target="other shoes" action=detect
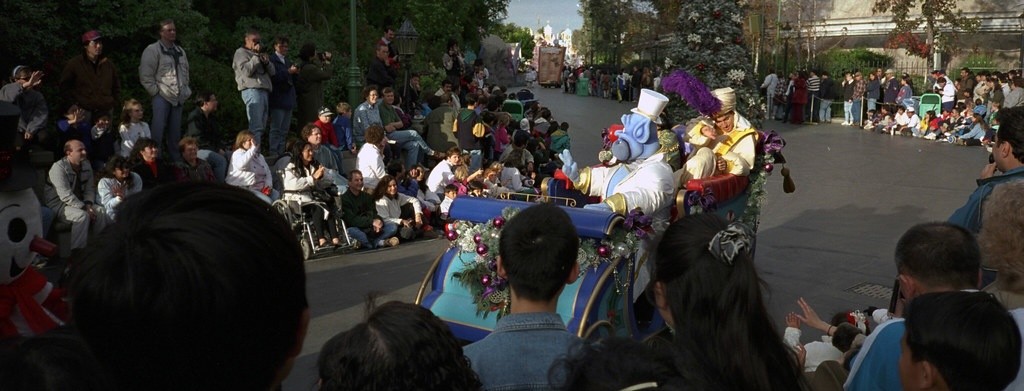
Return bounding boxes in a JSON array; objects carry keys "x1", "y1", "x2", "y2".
[
  {"x1": 825, "y1": 120, "x2": 831, "y2": 123},
  {"x1": 926, "y1": 133, "x2": 936, "y2": 140},
  {"x1": 841, "y1": 121, "x2": 848, "y2": 124},
  {"x1": 924, "y1": 132, "x2": 933, "y2": 138},
  {"x1": 632, "y1": 100, "x2": 637, "y2": 103},
  {"x1": 619, "y1": 99, "x2": 622, "y2": 103},
  {"x1": 848, "y1": 121, "x2": 853, "y2": 125},
  {"x1": 383, "y1": 237, "x2": 399, "y2": 247},
  {"x1": 331, "y1": 240, "x2": 339, "y2": 247},
  {"x1": 818, "y1": 121, "x2": 824, "y2": 124},
  {"x1": 319, "y1": 241, "x2": 333, "y2": 247}
]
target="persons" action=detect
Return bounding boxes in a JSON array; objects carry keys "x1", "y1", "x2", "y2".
[
  {"x1": 760, "y1": 68, "x2": 1024, "y2": 153},
  {"x1": 1, "y1": 107, "x2": 1024, "y2": 391},
  {"x1": 673, "y1": 87, "x2": 758, "y2": 190},
  {"x1": 0, "y1": 19, "x2": 571, "y2": 281},
  {"x1": 560, "y1": 64, "x2": 662, "y2": 104}
]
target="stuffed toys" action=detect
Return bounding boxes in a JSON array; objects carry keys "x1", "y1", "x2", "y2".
[
  {"x1": 558, "y1": 88, "x2": 685, "y2": 304},
  {"x1": 1, "y1": 101, "x2": 74, "y2": 339}
]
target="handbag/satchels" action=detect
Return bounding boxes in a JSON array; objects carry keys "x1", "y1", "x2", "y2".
[{"x1": 561, "y1": 85, "x2": 565, "y2": 93}]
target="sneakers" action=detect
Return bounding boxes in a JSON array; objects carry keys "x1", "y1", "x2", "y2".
[{"x1": 427, "y1": 149, "x2": 446, "y2": 160}]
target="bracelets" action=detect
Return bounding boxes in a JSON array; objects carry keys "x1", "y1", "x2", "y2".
[{"x1": 827, "y1": 325, "x2": 832, "y2": 336}]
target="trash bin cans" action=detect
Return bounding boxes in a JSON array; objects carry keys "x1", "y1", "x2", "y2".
[
  {"x1": 575, "y1": 78, "x2": 589, "y2": 96},
  {"x1": 919, "y1": 93, "x2": 941, "y2": 118},
  {"x1": 502, "y1": 100, "x2": 523, "y2": 123}
]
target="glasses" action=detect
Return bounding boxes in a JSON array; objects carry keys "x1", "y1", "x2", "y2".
[
  {"x1": 16, "y1": 77, "x2": 31, "y2": 82},
  {"x1": 115, "y1": 164, "x2": 129, "y2": 172}
]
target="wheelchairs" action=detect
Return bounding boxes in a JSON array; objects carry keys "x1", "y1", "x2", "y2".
[{"x1": 271, "y1": 190, "x2": 360, "y2": 261}]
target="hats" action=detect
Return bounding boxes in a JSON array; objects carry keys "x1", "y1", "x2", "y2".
[
  {"x1": 931, "y1": 69, "x2": 939, "y2": 73},
  {"x1": 663, "y1": 70, "x2": 737, "y2": 118},
  {"x1": 82, "y1": 31, "x2": 102, "y2": 42},
  {"x1": 885, "y1": 68, "x2": 894, "y2": 73},
  {"x1": 854, "y1": 72, "x2": 862, "y2": 75},
  {"x1": 318, "y1": 107, "x2": 334, "y2": 116},
  {"x1": 590, "y1": 67, "x2": 593, "y2": 69},
  {"x1": 905, "y1": 107, "x2": 914, "y2": 112}
]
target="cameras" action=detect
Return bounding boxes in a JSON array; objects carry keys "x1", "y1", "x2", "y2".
[
  {"x1": 318, "y1": 53, "x2": 333, "y2": 61},
  {"x1": 256, "y1": 42, "x2": 271, "y2": 53}
]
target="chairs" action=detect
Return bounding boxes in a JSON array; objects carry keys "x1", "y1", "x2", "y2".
[{"x1": 602, "y1": 124, "x2": 762, "y2": 217}]
